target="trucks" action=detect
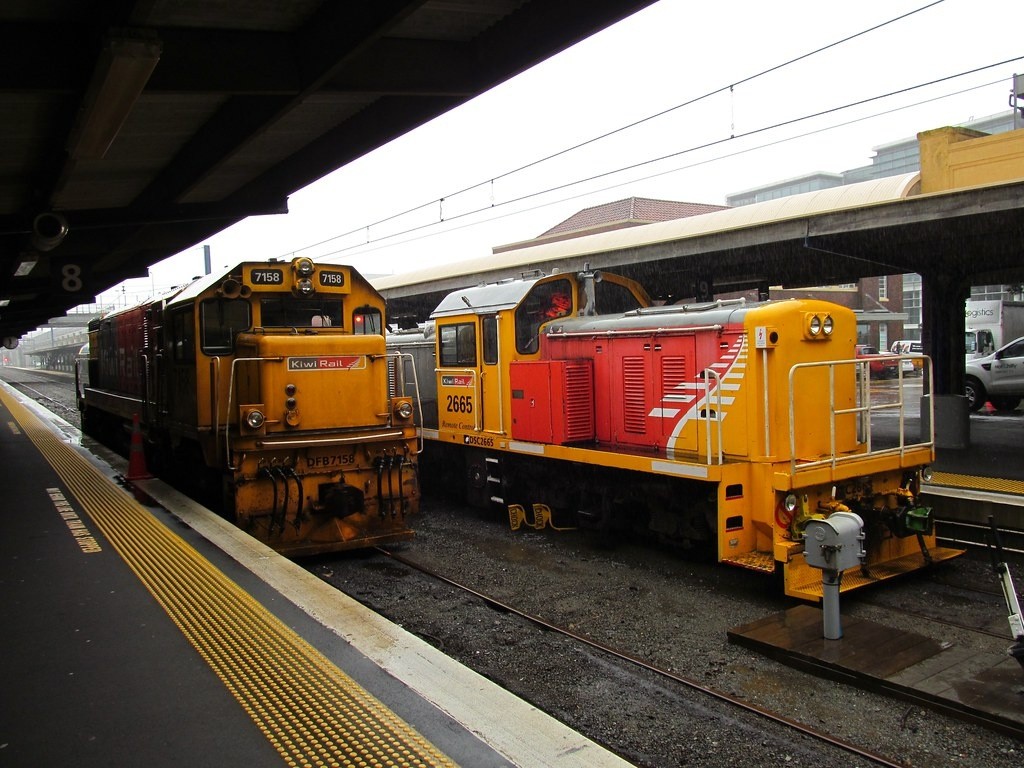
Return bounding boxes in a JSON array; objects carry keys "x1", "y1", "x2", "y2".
[{"x1": 965, "y1": 299, "x2": 1024, "y2": 360}]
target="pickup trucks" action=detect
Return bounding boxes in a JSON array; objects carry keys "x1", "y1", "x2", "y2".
[{"x1": 856, "y1": 340, "x2": 923, "y2": 376}]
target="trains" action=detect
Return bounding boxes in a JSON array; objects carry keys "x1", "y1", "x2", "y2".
[
  {"x1": 77, "y1": 264, "x2": 963, "y2": 603},
  {"x1": 74, "y1": 256, "x2": 424, "y2": 560}
]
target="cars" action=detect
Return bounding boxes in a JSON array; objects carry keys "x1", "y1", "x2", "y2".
[{"x1": 965, "y1": 336, "x2": 1024, "y2": 414}]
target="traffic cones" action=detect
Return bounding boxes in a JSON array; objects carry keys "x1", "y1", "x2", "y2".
[{"x1": 121, "y1": 413, "x2": 152, "y2": 480}]
